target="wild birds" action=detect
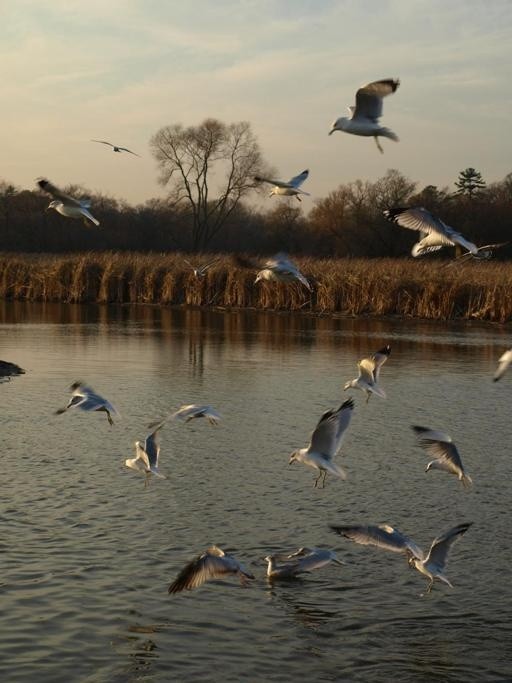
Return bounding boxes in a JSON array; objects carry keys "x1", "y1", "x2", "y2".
[
  {"x1": 288, "y1": 341, "x2": 393, "y2": 489},
  {"x1": 326, "y1": 522, "x2": 474, "y2": 592},
  {"x1": 254, "y1": 169, "x2": 311, "y2": 202},
  {"x1": 51, "y1": 378, "x2": 223, "y2": 488},
  {"x1": 181, "y1": 250, "x2": 322, "y2": 294},
  {"x1": 167, "y1": 546, "x2": 254, "y2": 593},
  {"x1": 37, "y1": 138, "x2": 138, "y2": 228},
  {"x1": 408, "y1": 423, "x2": 473, "y2": 490},
  {"x1": 327, "y1": 77, "x2": 401, "y2": 154},
  {"x1": 264, "y1": 546, "x2": 347, "y2": 581},
  {"x1": 491, "y1": 346, "x2": 512, "y2": 383},
  {"x1": 381, "y1": 206, "x2": 510, "y2": 269}
]
